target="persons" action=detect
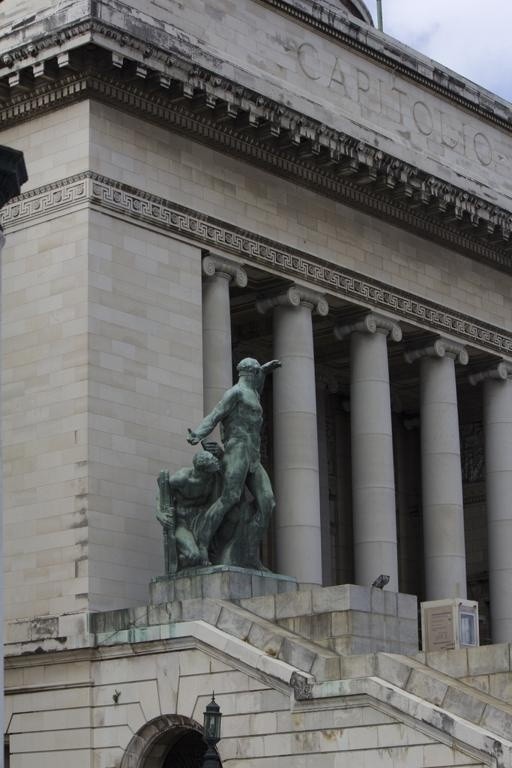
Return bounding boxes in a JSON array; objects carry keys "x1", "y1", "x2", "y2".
[
  {"x1": 143, "y1": 439, "x2": 222, "y2": 571},
  {"x1": 185, "y1": 354, "x2": 285, "y2": 577}
]
